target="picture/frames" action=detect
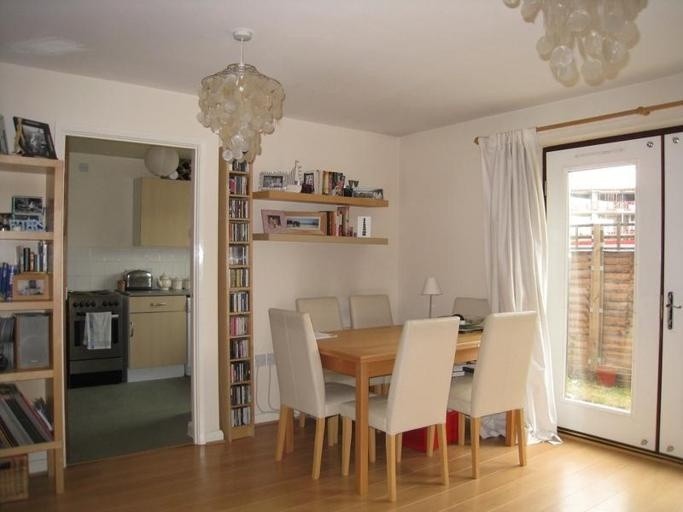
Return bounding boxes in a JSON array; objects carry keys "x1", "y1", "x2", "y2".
[
  {"x1": 282, "y1": 210, "x2": 327, "y2": 237},
  {"x1": 10, "y1": 272, "x2": 50, "y2": 302},
  {"x1": 11, "y1": 115, "x2": 57, "y2": 159},
  {"x1": 258, "y1": 170, "x2": 287, "y2": 192},
  {"x1": 260, "y1": 208, "x2": 287, "y2": 235}
]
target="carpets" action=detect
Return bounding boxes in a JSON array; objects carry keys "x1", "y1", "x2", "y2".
[{"x1": 64, "y1": 376, "x2": 195, "y2": 467}]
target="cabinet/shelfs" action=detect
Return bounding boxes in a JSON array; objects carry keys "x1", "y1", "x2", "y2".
[
  {"x1": 133, "y1": 175, "x2": 193, "y2": 250},
  {"x1": 249, "y1": 188, "x2": 390, "y2": 248},
  {"x1": 115, "y1": 290, "x2": 186, "y2": 383},
  {"x1": 216, "y1": 144, "x2": 255, "y2": 444},
  {"x1": 0, "y1": 151, "x2": 66, "y2": 506}
]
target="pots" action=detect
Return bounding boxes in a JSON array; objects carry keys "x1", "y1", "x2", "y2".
[{"x1": 123, "y1": 268, "x2": 154, "y2": 291}]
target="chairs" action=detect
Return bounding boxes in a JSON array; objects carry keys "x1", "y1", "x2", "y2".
[
  {"x1": 338, "y1": 314, "x2": 462, "y2": 505},
  {"x1": 450, "y1": 296, "x2": 491, "y2": 324},
  {"x1": 345, "y1": 292, "x2": 398, "y2": 436},
  {"x1": 423, "y1": 310, "x2": 542, "y2": 482},
  {"x1": 266, "y1": 305, "x2": 377, "y2": 484},
  {"x1": 293, "y1": 295, "x2": 366, "y2": 446}
]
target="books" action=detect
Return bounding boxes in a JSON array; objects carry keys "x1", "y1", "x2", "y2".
[
  {"x1": 303, "y1": 170, "x2": 346, "y2": 196},
  {"x1": 229, "y1": 244, "x2": 249, "y2": 265},
  {"x1": 229, "y1": 152, "x2": 248, "y2": 219},
  {"x1": 230, "y1": 223, "x2": 248, "y2": 242},
  {"x1": 231, "y1": 384, "x2": 252, "y2": 405},
  {"x1": 0, "y1": 241, "x2": 48, "y2": 301},
  {"x1": 0, "y1": 384, "x2": 53, "y2": 449},
  {"x1": 229, "y1": 269, "x2": 249, "y2": 287},
  {"x1": 231, "y1": 407, "x2": 250, "y2": 426},
  {"x1": 232, "y1": 292, "x2": 249, "y2": 312},
  {"x1": 230, "y1": 316, "x2": 248, "y2": 335},
  {"x1": 230, "y1": 338, "x2": 251, "y2": 382}
]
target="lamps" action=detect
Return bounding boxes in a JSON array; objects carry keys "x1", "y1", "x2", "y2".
[
  {"x1": 144, "y1": 148, "x2": 181, "y2": 177},
  {"x1": 501, "y1": 0, "x2": 653, "y2": 89},
  {"x1": 194, "y1": 28, "x2": 288, "y2": 165},
  {"x1": 419, "y1": 275, "x2": 442, "y2": 321}
]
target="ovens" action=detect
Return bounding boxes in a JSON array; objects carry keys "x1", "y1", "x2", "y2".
[{"x1": 65, "y1": 288, "x2": 125, "y2": 389}]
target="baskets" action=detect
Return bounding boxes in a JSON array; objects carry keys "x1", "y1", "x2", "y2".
[{"x1": 0, "y1": 453, "x2": 33, "y2": 505}]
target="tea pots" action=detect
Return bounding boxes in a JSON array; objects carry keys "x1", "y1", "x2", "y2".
[{"x1": 158, "y1": 272, "x2": 193, "y2": 291}]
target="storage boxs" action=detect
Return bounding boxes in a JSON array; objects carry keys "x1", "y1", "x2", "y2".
[{"x1": 401, "y1": 409, "x2": 460, "y2": 454}]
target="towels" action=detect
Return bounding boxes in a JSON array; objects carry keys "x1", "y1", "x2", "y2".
[{"x1": 82, "y1": 311, "x2": 113, "y2": 351}]
